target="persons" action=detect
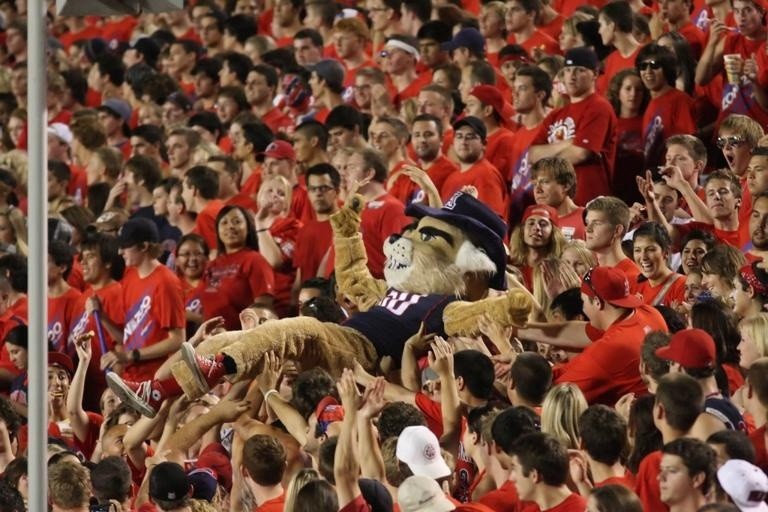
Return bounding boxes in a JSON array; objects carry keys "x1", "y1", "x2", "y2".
[{"x1": 0, "y1": 0, "x2": 767, "y2": 511}]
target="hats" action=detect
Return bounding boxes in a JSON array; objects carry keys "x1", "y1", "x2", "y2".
[
  {"x1": 580, "y1": 266, "x2": 643, "y2": 309},
  {"x1": 453, "y1": 83, "x2": 508, "y2": 142},
  {"x1": 564, "y1": 46, "x2": 600, "y2": 72},
  {"x1": 302, "y1": 59, "x2": 345, "y2": 88},
  {"x1": 47, "y1": 97, "x2": 131, "y2": 149},
  {"x1": 396, "y1": 425, "x2": 452, "y2": 480},
  {"x1": 254, "y1": 138, "x2": 296, "y2": 163},
  {"x1": 438, "y1": 27, "x2": 485, "y2": 53},
  {"x1": 655, "y1": 328, "x2": 718, "y2": 368},
  {"x1": 46, "y1": 351, "x2": 73, "y2": 370},
  {"x1": 717, "y1": 458, "x2": 768, "y2": 512},
  {"x1": 148, "y1": 440, "x2": 234, "y2": 503},
  {"x1": 397, "y1": 475, "x2": 456, "y2": 512},
  {"x1": 90, "y1": 205, "x2": 160, "y2": 249}
]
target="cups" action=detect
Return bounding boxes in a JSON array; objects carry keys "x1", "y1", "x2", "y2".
[{"x1": 723, "y1": 53, "x2": 741, "y2": 85}]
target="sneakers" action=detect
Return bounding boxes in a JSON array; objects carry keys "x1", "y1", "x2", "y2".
[
  {"x1": 104, "y1": 372, "x2": 168, "y2": 420},
  {"x1": 180, "y1": 341, "x2": 227, "y2": 391}
]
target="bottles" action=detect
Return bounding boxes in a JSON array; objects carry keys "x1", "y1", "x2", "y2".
[{"x1": 74, "y1": 187, "x2": 82, "y2": 208}]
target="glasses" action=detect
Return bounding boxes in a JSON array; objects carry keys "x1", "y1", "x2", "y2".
[
  {"x1": 637, "y1": 59, "x2": 665, "y2": 70},
  {"x1": 716, "y1": 134, "x2": 748, "y2": 149},
  {"x1": 583, "y1": 268, "x2": 604, "y2": 304}
]
